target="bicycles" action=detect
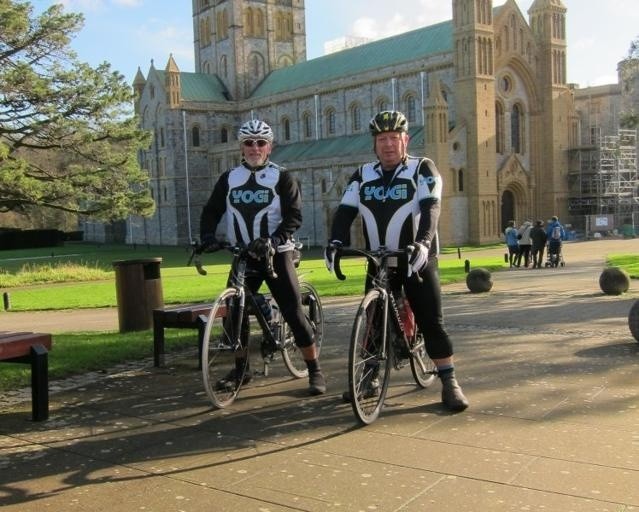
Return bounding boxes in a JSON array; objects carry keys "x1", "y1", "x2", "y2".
[
  {"x1": 185, "y1": 237, "x2": 324, "y2": 409},
  {"x1": 327, "y1": 238, "x2": 441, "y2": 426}
]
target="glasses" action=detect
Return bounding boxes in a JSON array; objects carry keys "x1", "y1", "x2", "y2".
[{"x1": 244, "y1": 140, "x2": 266, "y2": 146}]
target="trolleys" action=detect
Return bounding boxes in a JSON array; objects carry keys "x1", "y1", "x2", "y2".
[{"x1": 545, "y1": 243, "x2": 565, "y2": 268}]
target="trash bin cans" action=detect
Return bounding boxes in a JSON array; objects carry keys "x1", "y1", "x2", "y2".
[{"x1": 111, "y1": 257, "x2": 163, "y2": 332}]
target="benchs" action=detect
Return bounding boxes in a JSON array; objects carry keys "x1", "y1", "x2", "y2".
[
  {"x1": 0, "y1": 329, "x2": 52, "y2": 421},
  {"x1": 151, "y1": 291, "x2": 317, "y2": 369}
]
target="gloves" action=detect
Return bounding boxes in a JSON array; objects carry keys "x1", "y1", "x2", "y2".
[
  {"x1": 200, "y1": 233, "x2": 220, "y2": 253},
  {"x1": 249, "y1": 238, "x2": 273, "y2": 255},
  {"x1": 324, "y1": 239, "x2": 344, "y2": 271},
  {"x1": 408, "y1": 240, "x2": 431, "y2": 272}
]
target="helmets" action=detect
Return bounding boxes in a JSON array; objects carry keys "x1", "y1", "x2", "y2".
[
  {"x1": 368, "y1": 110, "x2": 409, "y2": 137},
  {"x1": 237, "y1": 120, "x2": 274, "y2": 143}
]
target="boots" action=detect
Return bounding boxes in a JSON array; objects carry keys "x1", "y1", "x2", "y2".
[
  {"x1": 438, "y1": 368, "x2": 469, "y2": 410},
  {"x1": 305, "y1": 358, "x2": 326, "y2": 394},
  {"x1": 216, "y1": 355, "x2": 251, "y2": 389},
  {"x1": 343, "y1": 363, "x2": 380, "y2": 403}
]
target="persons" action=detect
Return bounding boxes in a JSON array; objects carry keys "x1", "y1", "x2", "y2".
[
  {"x1": 323, "y1": 110, "x2": 470, "y2": 413},
  {"x1": 199, "y1": 120, "x2": 326, "y2": 397},
  {"x1": 503, "y1": 214, "x2": 565, "y2": 269}
]
